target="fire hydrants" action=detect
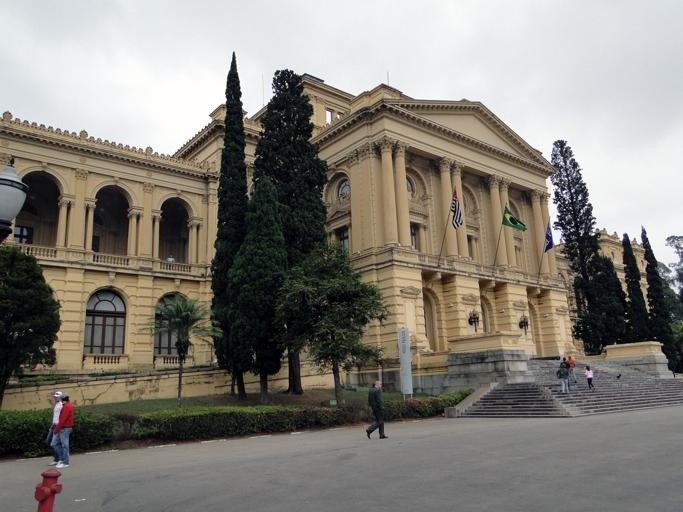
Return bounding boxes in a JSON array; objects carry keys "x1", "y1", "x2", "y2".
[{"x1": 34, "y1": 468, "x2": 63, "y2": 512}]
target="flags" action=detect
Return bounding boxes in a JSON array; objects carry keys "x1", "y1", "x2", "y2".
[
  {"x1": 501, "y1": 206, "x2": 528, "y2": 232},
  {"x1": 543, "y1": 224, "x2": 553, "y2": 253},
  {"x1": 449, "y1": 190, "x2": 463, "y2": 230}
]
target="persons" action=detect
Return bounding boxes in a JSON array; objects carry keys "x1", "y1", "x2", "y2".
[
  {"x1": 584, "y1": 366, "x2": 595, "y2": 392},
  {"x1": 50, "y1": 393, "x2": 74, "y2": 468},
  {"x1": 558, "y1": 356, "x2": 577, "y2": 393},
  {"x1": 46, "y1": 391, "x2": 63, "y2": 466},
  {"x1": 165, "y1": 254, "x2": 175, "y2": 270},
  {"x1": 366, "y1": 381, "x2": 389, "y2": 439}
]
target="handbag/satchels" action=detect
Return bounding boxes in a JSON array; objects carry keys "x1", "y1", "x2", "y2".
[{"x1": 556, "y1": 370, "x2": 561, "y2": 378}]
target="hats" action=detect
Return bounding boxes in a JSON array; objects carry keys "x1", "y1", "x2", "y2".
[{"x1": 52, "y1": 391, "x2": 69, "y2": 398}]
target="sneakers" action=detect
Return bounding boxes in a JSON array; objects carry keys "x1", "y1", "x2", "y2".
[
  {"x1": 575, "y1": 380, "x2": 577, "y2": 383},
  {"x1": 48, "y1": 461, "x2": 69, "y2": 468}
]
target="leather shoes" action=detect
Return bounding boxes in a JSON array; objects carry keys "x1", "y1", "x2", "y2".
[
  {"x1": 380, "y1": 436, "x2": 388, "y2": 439},
  {"x1": 366, "y1": 430, "x2": 370, "y2": 439}
]
